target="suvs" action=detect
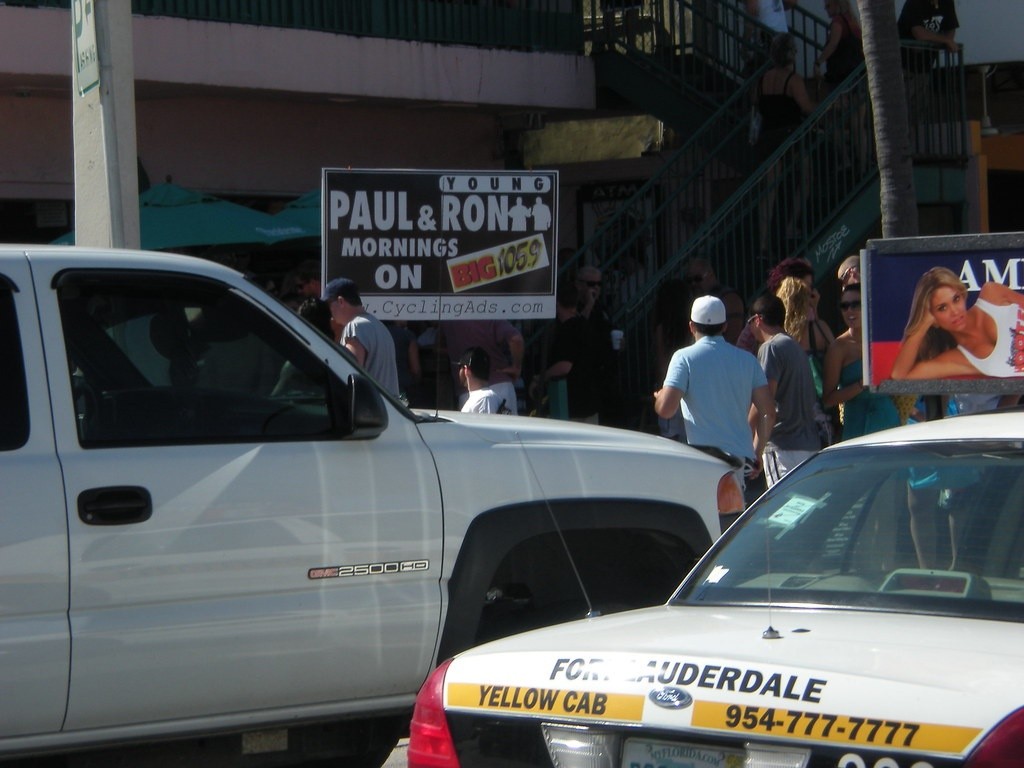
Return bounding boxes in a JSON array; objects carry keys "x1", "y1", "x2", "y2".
[{"x1": 1, "y1": 245, "x2": 755, "y2": 765}]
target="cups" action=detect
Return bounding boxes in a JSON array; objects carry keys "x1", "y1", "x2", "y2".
[{"x1": 610, "y1": 330, "x2": 624, "y2": 350}]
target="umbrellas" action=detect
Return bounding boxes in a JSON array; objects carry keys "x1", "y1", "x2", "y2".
[
  {"x1": 273, "y1": 186, "x2": 321, "y2": 232},
  {"x1": 49, "y1": 183, "x2": 321, "y2": 250}
]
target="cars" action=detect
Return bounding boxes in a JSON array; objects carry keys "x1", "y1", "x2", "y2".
[{"x1": 407, "y1": 411, "x2": 1024, "y2": 768}]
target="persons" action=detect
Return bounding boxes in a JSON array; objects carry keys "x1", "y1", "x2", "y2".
[
  {"x1": 740, "y1": 0, "x2": 959, "y2": 257},
  {"x1": 651, "y1": 298, "x2": 693, "y2": 442},
  {"x1": 837, "y1": 255, "x2": 860, "y2": 288},
  {"x1": 907, "y1": 391, "x2": 1024, "y2": 572},
  {"x1": 320, "y1": 278, "x2": 399, "y2": 398},
  {"x1": 270, "y1": 299, "x2": 336, "y2": 397},
  {"x1": 822, "y1": 283, "x2": 902, "y2": 441},
  {"x1": 600, "y1": 0, "x2": 644, "y2": 51},
  {"x1": 891, "y1": 266, "x2": 1024, "y2": 381},
  {"x1": 451, "y1": 346, "x2": 511, "y2": 414},
  {"x1": 233, "y1": 259, "x2": 626, "y2": 427},
  {"x1": 748, "y1": 295, "x2": 821, "y2": 488},
  {"x1": 654, "y1": 295, "x2": 777, "y2": 509},
  {"x1": 736, "y1": 258, "x2": 814, "y2": 358},
  {"x1": 776, "y1": 276, "x2": 843, "y2": 450},
  {"x1": 687, "y1": 257, "x2": 745, "y2": 345}
]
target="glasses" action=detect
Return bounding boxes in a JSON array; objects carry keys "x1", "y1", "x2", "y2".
[
  {"x1": 586, "y1": 280, "x2": 602, "y2": 286},
  {"x1": 840, "y1": 300, "x2": 861, "y2": 311}
]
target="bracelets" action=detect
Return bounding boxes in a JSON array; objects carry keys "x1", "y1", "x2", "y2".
[{"x1": 858, "y1": 381, "x2": 865, "y2": 392}]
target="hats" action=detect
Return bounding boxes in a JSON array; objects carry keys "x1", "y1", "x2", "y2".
[
  {"x1": 691, "y1": 294, "x2": 726, "y2": 325},
  {"x1": 557, "y1": 286, "x2": 580, "y2": 307},
  {"x1": 460, "y1": 347, "x2": 491, "y2": 379},
  {"x1": 318, "y1": 277, "x2": 359, "y2": 301}
]
